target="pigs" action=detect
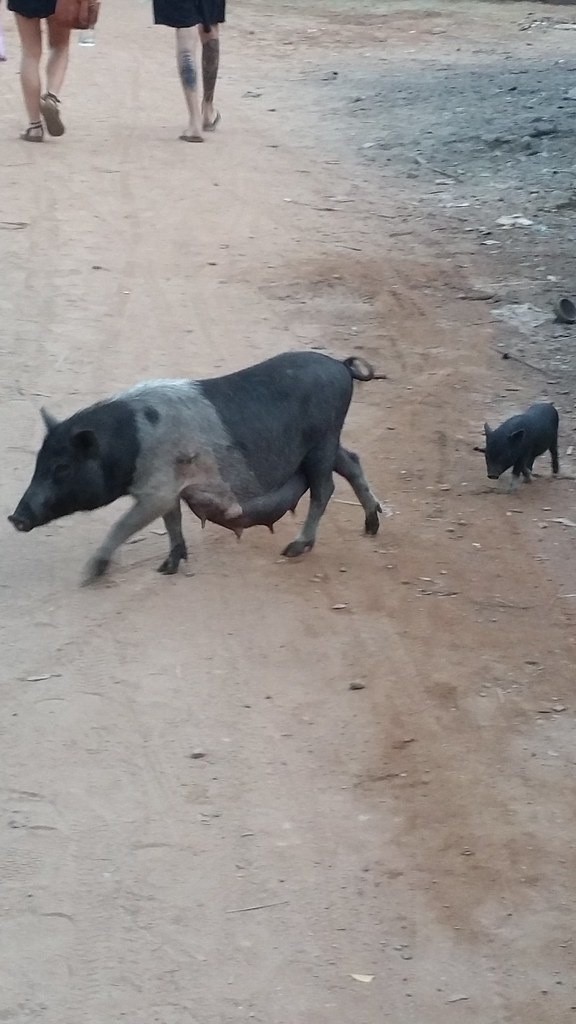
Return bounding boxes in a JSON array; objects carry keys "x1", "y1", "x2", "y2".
[
  {"x1": 484, "y1": 402, "x2": 559, "y2": 486},
  {"x1": 6, "y1": 351, "x2": 381, "y2": 587}
]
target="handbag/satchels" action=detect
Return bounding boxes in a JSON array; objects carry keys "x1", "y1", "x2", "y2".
[{"x1": 54, "y1": 1, "x2": 100, "y2": 29}]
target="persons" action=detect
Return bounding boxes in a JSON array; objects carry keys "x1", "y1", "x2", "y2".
[
  {"x1": 151, "y1": 0, "x2": 228, "y2": 143},
  {"x1": 4, "y1": 0, "x2": 73, "y2": 144}
]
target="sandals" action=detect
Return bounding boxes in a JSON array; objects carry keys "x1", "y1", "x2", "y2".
[
  {"x1": 40, "y1": 93, "x2": 65, "y2": 137},
  {"x1": 20, "y1": 120, "x2": 45, "y2": 142}
]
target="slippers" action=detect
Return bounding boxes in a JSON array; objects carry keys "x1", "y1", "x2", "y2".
[
  {"x1": 203, "y1": 109, "x2": 222, "y2": 131},
  {"x1": 179, "y1": 128, "x2": 204, "y2": 143}
]
